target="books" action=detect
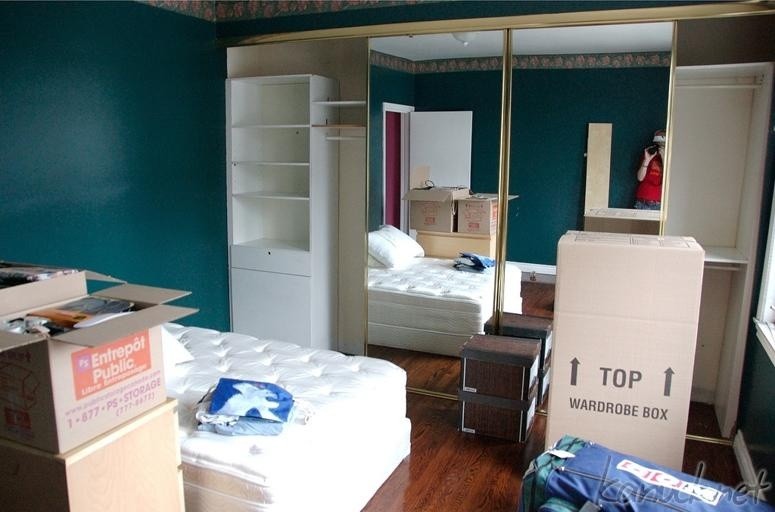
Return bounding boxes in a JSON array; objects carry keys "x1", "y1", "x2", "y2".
[{"x1": 24, "y1": 296, "x2": 136, "y2": 328}]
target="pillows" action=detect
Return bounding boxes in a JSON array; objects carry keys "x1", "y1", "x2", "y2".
[{"x1": 366, "y1": 223, "x2": 426, "y2": 271}]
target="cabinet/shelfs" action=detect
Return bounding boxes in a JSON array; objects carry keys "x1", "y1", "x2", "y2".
[
  {"x1": 224, "y1": 73, "x2": 367, "y2": 350},
  {"x1": 411, "y1": 229, "x2": 499, "y2": 261},
  {"x1": 1, "y1": 395, "x2": 189, "y2": 512},
  {"x1": 663, "y1": 57, "x2": 774, "y2": 442}
]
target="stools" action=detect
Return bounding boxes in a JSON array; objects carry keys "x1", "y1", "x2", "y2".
[
  {"x1": 483, "y1": 307, "x2": 553, "y2": 410},
  {"x1": 456, "y1": 330, "x2": 542, "y2": 443}
]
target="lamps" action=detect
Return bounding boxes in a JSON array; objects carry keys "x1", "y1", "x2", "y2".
[{"x1": 452, "y1": 31, "x2": 479, "y2": 46}]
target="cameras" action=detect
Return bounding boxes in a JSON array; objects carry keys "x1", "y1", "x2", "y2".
[{"x1": 648, "y1": 143, "x2": 660, "y2": 155}]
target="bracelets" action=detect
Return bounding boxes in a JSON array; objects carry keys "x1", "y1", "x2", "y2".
[{"x1": 641, "y1": 164, "x2": 648, "y2": 169}]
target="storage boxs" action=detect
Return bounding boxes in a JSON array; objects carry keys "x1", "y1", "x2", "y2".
[
  {"x1": 0, "y1": 253, "x2": 129, "y2": 320},
  {"x1": 544, "y1": 228, "x2": 707, "y2": 471},
  {"x1": 0, "y1": 282, "x2": 201, "y2": 454},
  {"x1": 452, "y1": 191, "x2": 522, "y2": 236},
  {"x1": 399, "y1": 181, "x2": 470, "y2": 233}
]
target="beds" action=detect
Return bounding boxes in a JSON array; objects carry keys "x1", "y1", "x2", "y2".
[
  {"x1": 367, "y1": 222, "x2": 525, "y2": 359},
  {"x1": 159, "y1": 322, "x2": 414, "y2": 512}
]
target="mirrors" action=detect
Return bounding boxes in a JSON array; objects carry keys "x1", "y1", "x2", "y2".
[{"x1": 367, "y1": 18, "x2": 679, "y2": 418}]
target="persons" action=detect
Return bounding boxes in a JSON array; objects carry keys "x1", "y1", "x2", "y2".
[{"x1": 634, "y1": 127, "x2": 667, "y2": 210}]
